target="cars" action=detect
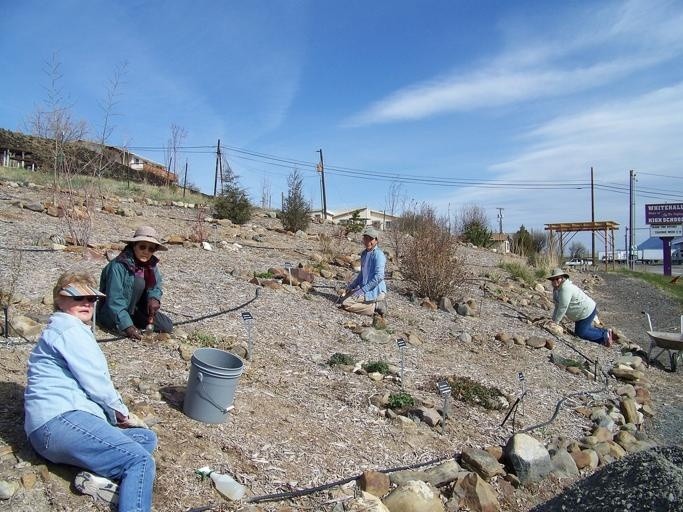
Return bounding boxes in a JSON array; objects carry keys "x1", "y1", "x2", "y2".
[{"x1": 565, "y1": 258, "x2": 593, "y2": 266}]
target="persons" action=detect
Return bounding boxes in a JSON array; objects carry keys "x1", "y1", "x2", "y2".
[
  {"x1": 21, "y1": 266, "x2": 158, "y2": 512},
  {"x1": 542, "y1": 267, "x2": 613, "y2": 348},
  {"x1": 336, "y1": 226, "x2": 389, "y2": 320},
  {"x1": 88, "y1": 226, "x2": 174, "y2": 344}
]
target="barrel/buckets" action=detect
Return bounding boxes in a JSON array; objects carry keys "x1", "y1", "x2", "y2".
[{"x1": 183, "y1": 347, "x2": 244, "y2": 423}]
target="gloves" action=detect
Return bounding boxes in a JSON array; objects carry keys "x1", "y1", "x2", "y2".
[
  {"x1": 125, "y1": 325, "x2": 141, "y2": 341},
  {"x1": 146, "y1": 298, "x2": 160, "y2": 315},
  {"x1": 116, "y1": 413, "x2": 148, "y2": 429},
  {"x1": 345, "y1": 286, "x2": 353, "y2": 293},
  {"x1": 351, "y1": 288, "x2": 364, "y2": 302}
]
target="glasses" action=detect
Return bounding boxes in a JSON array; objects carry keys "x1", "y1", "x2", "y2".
[
  {"x1": 73, "y1": 296, "x2": 97, "y2": 302},
  {"x1": 138, "y1": 245, "x2": 155, "y2": 252},
  {"x1": 551, "y1": 277, "x2": 559, "y2": 282}
]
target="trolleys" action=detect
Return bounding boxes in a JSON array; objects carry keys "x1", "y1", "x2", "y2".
[{"x1": 640, "y1": 310, "x2": 683, "y2": 372}]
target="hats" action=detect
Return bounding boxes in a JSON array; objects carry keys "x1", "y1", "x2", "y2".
[
  {"x1": 360, "y1": 229, "x2": 378, "y2": 240},
  {"x1": 545, "y1": 268, "x2": 568, "y2": 281},
  {"x1": 58, "y1": 282, "x2": 106, "y2": 297},
  {"x1": 118, "y1": 226, "x2": 168, "y2": 250}
]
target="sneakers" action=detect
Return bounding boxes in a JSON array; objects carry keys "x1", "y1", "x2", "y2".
[
  {"x1": 375, "y1": 302, "x2": 386, "y2": 320},
  {"x1": 74, "y1": 471, "x2": 120, "y2": 505},
  {"x1": 606, "y1": 328, "x2": 612, "y2": 347}
]
